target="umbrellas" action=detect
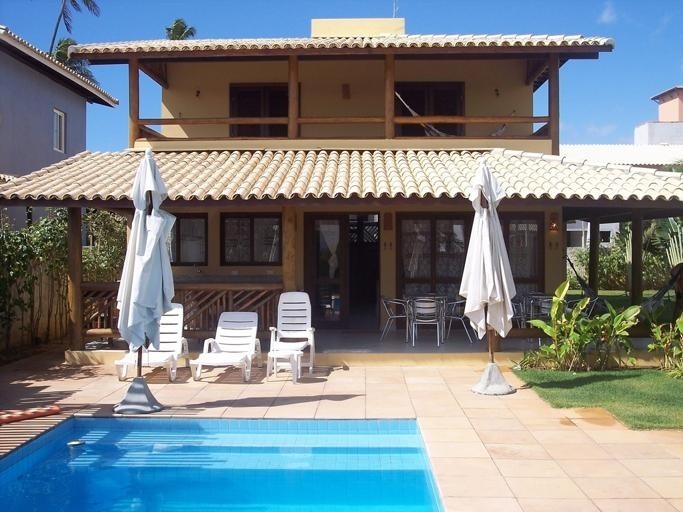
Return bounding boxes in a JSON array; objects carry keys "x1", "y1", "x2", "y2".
[
  {"x1": 114, "y1": 145, "x2": 177, "y2": 378},
  {"x1": 455, "y1": 154, "x2": 518, "y2": 363}
]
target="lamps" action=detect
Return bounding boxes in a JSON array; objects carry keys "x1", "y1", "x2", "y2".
[{"x1": 549, "y1": 213, "x2": 559, "y2": 233}]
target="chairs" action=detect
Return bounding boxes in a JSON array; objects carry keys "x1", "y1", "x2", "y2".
[
  {"x1": 379, "y1": 294, "x2": 473, "y2": 348},
  {"x1": 512, "y1": 292, "x2": 598, "y2": 329},
  {"x1": 114, "y1": 291, "x2": 315, "y2": 385}
]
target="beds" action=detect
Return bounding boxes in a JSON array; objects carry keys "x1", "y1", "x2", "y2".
[
  {"x1": 568, "y1": 254, "x2": 683, "y2": 317},
  {"x1": 395, "y1": 91, "x2": 519, "y2": 138}
]
